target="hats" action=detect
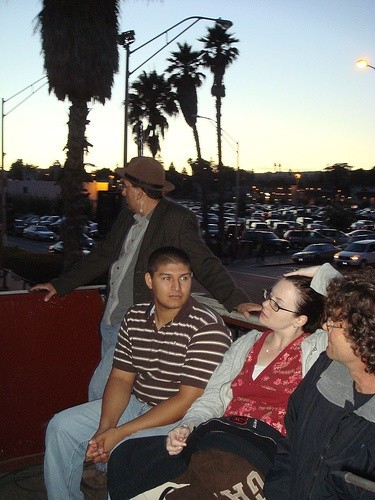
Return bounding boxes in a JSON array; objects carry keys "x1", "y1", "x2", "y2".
[{"x1": 114, "y1": 156, "x2": 175, "y2": 191}]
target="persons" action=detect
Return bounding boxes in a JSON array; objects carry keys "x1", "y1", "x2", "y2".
[
  {"x1": 28, "y1": 156, "x2": 263, "y2": 492},
  {"x1": 262, "y1": 270, "x2": 375, "y2": 500},
  {"x1": 106, "y1": 262, "x2": 346, "y2": 500},
  {"x1": 43, "y1": 245, "x2": 235, "y2": 500}
]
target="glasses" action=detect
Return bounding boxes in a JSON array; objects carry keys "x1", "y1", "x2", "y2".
[
  {"x1": 263, "y1": 288, "x2": 303, "y2": 315},
  {"x1": 326, "y1": 317, "x2": 349, "y2": 333},
  {"x1": 121, "y1": 185, "x2": 142, "y2": 189}
]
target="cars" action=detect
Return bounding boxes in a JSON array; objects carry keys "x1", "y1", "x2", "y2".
[
  {"x1": 290, "y1": 243, "x2": 336, "y2": 266},
  {"x1": 13, "y1": 213, "x2": 100, "y2": 255},
  {"x1": 314, "y1": 228, "x2": 350, "y2": 243},
  {"x1": 174, "y1": 201, "x2": 375, "y2": 243},
  {"x1": 239, "y1": 230, "x2": 290, "y2": 255},
  {"x1": 333, "y1": 239, "x2": 375, "y2": 269},
  {"x1": 23, "y1": 225, "x2": 57, "y2": 241},
  {"x1": 283, "y1": 230, "x2": 332, "y2": 245}
]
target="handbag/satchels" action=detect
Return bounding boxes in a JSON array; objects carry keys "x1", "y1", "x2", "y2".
[
  {"x1": 182, "y1": 415, "x2": 290, "y2": 483},
  {"x1": 158, "y1": 449, "x2": 267, "y2": 500}
]
